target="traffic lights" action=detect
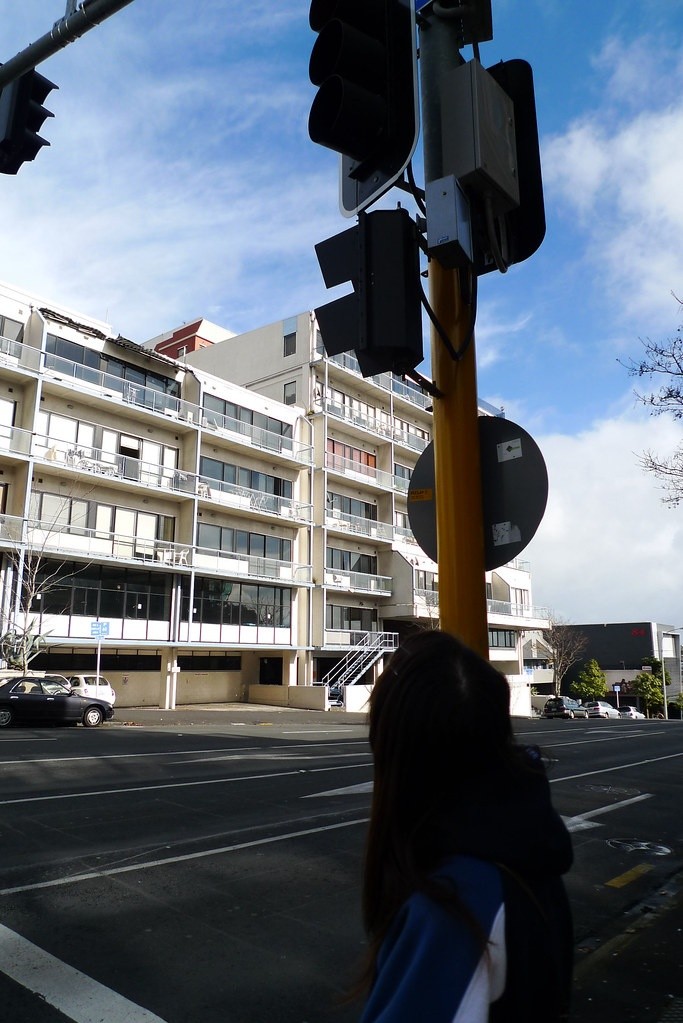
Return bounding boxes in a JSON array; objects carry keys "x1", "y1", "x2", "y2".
[
  {"x1": 303, "y1": 1, "x2": 419, "y2": 219},
  {"x1": 0, "y1": 63, "x2": 55, "y2": 175},
  {"x1": 461, "y1": 56, "x2": 547, "y2": 277},
  {"x1": 312, "y1": 205, "x2": 425, "y2": 374}
]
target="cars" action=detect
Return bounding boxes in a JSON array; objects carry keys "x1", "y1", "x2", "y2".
[
  {"x1": 616, "y1": 706, "x2": 646, "y2": 720},
  {"x1": 543, "y1": 698, "x2": 589, "y2": 719},
  {"x1": 583, "y1": 701, "x2": 623, "y2": 718},
  {"x1": 66, "y1": 675, "x2": 115, "y2": 706},
  {"x1": 43, "y1": 673, "x2": 71, "y2": 696},
  {"x1": 0, "y1": 677, "x2": 114, "y2": 727}
]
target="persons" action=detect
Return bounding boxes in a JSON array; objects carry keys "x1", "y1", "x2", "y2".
[{"x1": 349, "y1": 632, "x2": 580, "y2": 1022}]
